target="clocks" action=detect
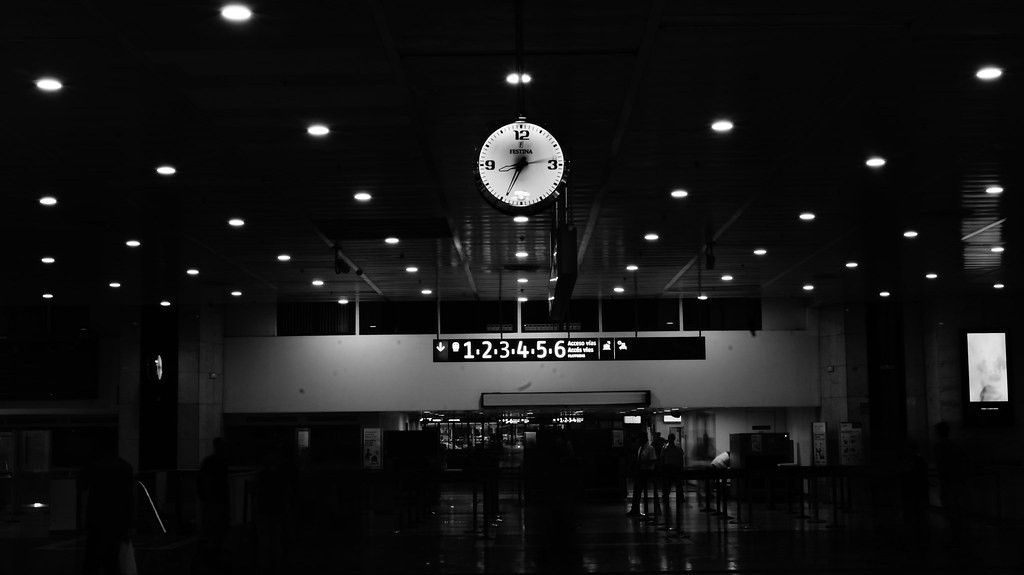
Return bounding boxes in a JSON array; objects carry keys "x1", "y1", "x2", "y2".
[{"x1": 471, "y1": 114, "x2": 571, "y2": 216}]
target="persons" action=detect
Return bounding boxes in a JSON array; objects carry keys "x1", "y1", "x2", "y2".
[
  {"x1": 196, "y1": 437, "x2": 232, "y2": 561},
  {"x1": 933, "y1": 423, "x2": 971, "y2": 547},
  {"x1": 79, "y1": 435, "x2": 134, "y2": 575},
  {"x1": 895, "y1": 440, "x2": 930, "y2": 551},
  {"x1": 626, "y1": 432, "x2": 687, "y2": 537}
]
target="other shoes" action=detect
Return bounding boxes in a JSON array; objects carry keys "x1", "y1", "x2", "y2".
[{"x1": 625, "y1": 511, "x2": 641, "y2": 516}]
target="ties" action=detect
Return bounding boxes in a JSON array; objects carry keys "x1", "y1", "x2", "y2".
[{"x1": 639, "y1": 447, "x2": 644, "y2": 461}]
